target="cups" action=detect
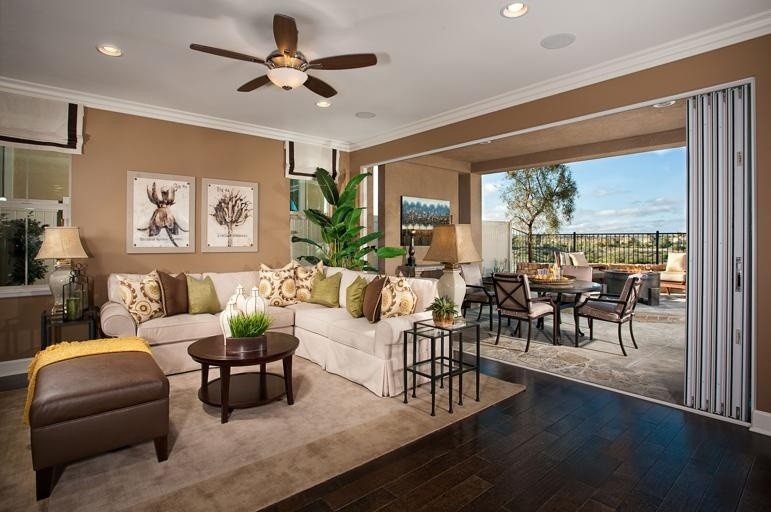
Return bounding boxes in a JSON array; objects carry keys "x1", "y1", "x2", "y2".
[{"x1": 537, "y1": 264, "x2": 564, "y2": 282}]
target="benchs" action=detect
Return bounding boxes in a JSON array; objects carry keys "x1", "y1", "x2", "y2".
[{"x1": 27, "y1": 340, "x2": 174, "y2": 503}]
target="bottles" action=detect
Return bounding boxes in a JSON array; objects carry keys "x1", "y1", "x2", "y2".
[{"x1": 219, "y1": 284, "x2": 266, "y2": 347}]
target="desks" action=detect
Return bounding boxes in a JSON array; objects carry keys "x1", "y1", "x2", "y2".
[
  {"x1": 184, "y1": 329, "x2": 302, "y2": 425},
  {"x1": 400, "y1": 316, "x2": 483, "y2": 418},
  {"x1": 37, "y1": 308, "x2": 101, "y2": 349}
]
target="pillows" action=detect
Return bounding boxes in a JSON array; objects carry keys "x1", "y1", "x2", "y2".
[
  {"x1": 256, "y1": 257, "x2": 420, "y2": 326},
  {"x1": 114, "y1": 259, "x2": 226, "y2": 322}
]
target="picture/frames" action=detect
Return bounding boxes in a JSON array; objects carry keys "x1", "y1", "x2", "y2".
[
  {"x1": 200, "y1": 176, "x2": 261, "y2": 254},
  {"x1": 124, "y1": 169, "x2": 198, "y2": 254}
]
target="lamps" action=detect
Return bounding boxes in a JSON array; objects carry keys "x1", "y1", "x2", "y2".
[
  {"x1": 422, "y1": 223, "x2": 486, "y2": 322},
  {"x1": 263, "y1": 62, "x2": 311, "y2": 91},
  {"x1": 33, "y1": 226, "x2": 95, "y2": 318}
]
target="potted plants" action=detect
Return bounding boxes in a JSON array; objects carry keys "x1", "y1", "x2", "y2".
[
  {"x1": 423, "y1": 295, "x2": 462, "y2": 326},
  {"x1": 225, "y1": 308, "x2": 272, "y2": 359}
]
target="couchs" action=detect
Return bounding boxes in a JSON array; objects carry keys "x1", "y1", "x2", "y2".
[{"x1": 98, "y1": 257, "x2": 463, "y2": 402}]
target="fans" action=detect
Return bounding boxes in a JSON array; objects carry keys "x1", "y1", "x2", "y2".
[{"x1": 187, "y1": 12, "x2": 379, "y2": 98}]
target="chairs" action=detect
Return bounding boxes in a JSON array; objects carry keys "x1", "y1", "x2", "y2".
[{"x1": 459, "y1": 244, "x2": 689, "y2": 360}]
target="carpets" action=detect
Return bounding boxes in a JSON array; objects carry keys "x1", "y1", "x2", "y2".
[{"x1": 1, "y1": 340, "x2": 532, "y2": 511}]
